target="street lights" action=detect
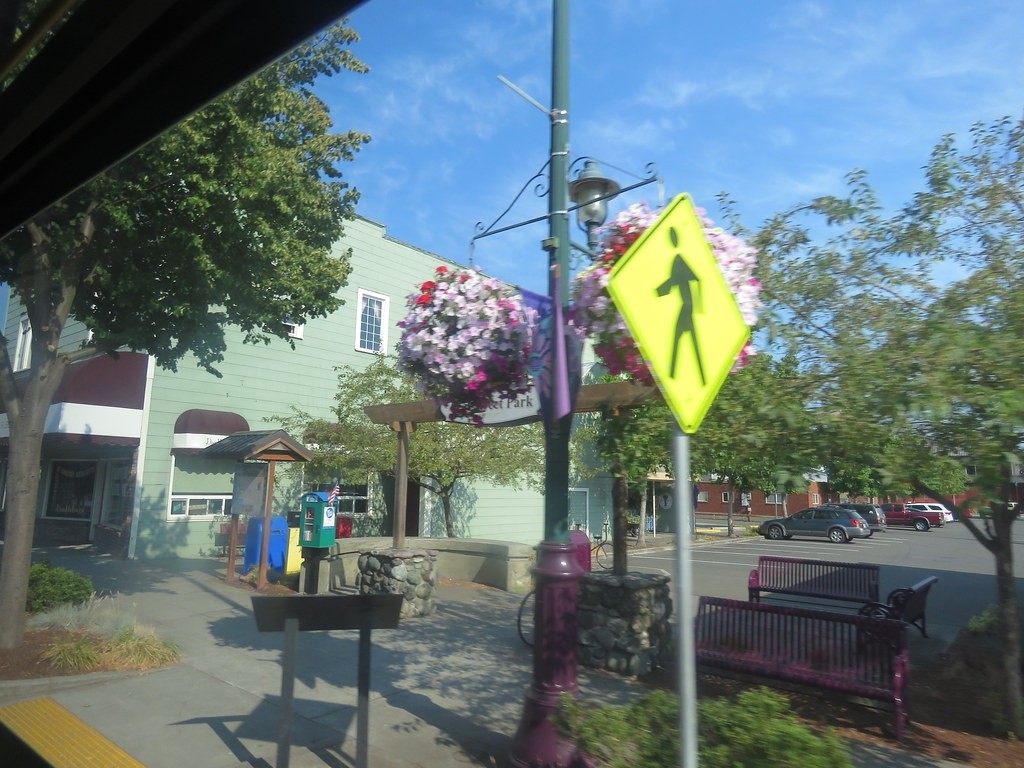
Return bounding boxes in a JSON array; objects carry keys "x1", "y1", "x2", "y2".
[{"x1": 468, "y1": 0, "x2": 660, "y2": 768}]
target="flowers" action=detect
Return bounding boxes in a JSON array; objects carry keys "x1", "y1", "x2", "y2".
[
  {"x1": 394, "y1": 266, "x2": 537, "y2": 428},
  {"x1": 567, "y1": 202, "x2": 763, "y2": 399}
]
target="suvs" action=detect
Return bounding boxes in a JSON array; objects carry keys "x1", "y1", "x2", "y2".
[{"x1": 826, "y1": 503, "x2": 887, "y2": 535}]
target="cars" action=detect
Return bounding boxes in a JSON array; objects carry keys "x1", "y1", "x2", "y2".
[
  {"x1": 1008, "y1": 502, "x2": 1018, "y2": 511},
  {"x1": 756, "y1": 505, "x2": 870, "y2": 542},
  {"x1": 906, "y1": 504, "x2": 953, "y2": 522}
]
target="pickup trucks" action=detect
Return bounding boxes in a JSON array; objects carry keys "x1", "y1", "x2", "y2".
[{"x1": 876, "y1": 503, "x2": 944, "y2": 531}]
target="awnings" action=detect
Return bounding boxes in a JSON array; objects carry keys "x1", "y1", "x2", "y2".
[
  {"x1": 1, "y1": 352, "x2": 149, "y2": 446},
  {"x1": 172, "y1": 410, "x2": 250, "y2": 455}
]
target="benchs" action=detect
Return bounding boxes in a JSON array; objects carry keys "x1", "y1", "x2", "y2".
[
  {"x1": 220, "y1": 523, "x2": 248, "y2": 558},
  {"x1": 748, "y1": 556, "x2": 880, "y2": 603},
  {"x1": 694, "y1": 596, "x2": 912, "y2": 742},
  {"x1": 856, "y1": 576, "x2": 938, "y2": 654}
]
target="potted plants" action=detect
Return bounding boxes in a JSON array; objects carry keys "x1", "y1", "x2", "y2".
[{"x1": 626, "y1": 515, "x2": 641, "y2": 531}]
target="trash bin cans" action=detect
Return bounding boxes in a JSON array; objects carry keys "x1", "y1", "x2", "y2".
[
  {"x1": 284, "y1": 526, "x2": 305, "y2": 576},
  {"x1": 337, "y1": 515, "x2": 352, "y2": 539},
  {"x1": 287, "y1": 510, "x2": 301, "y2": 528},
  {"x1": 243, "y1": 516, "x2": 288, "y2": 577}
]
